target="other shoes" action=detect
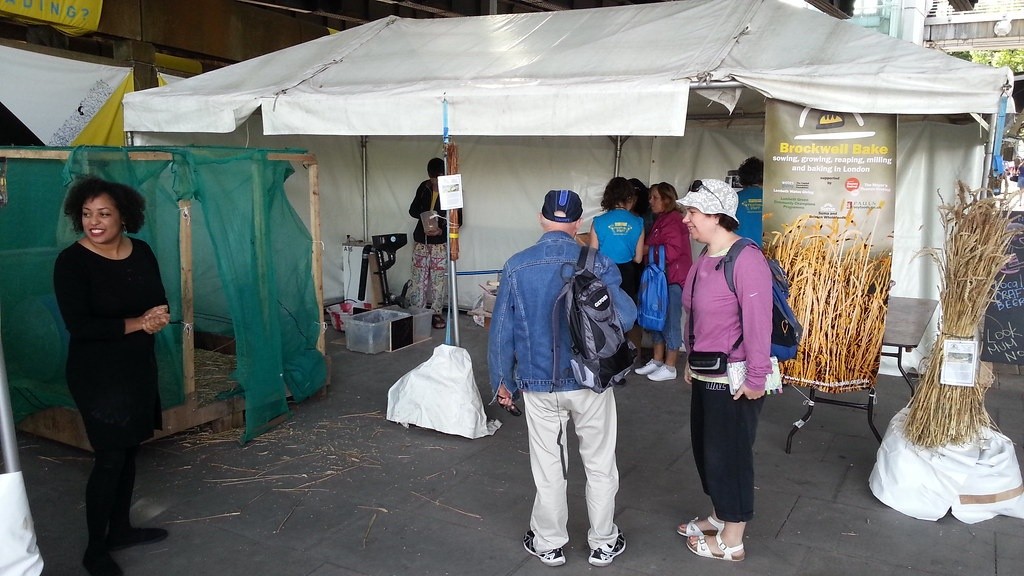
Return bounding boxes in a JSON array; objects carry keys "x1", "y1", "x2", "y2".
[
  {"x1": 83, "y1": 548, "x2": 124, "y2": 576},
  {"x1": 108, "y1": 526, "x2": 168, "y2": 550}
]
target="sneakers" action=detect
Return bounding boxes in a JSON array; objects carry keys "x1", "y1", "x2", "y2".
[
  {"x1": 588, "y1": 529, "x2": 626, "y2": 566},
  {"x1": 634, "y1": 359, "x2": 664, "y2": 375},
  {"x1": 522, "y1": 528, "x2": 566, "y2": 567},
  {"x1": 646, "y1": 365, "x2": 677, "y2": 381}
]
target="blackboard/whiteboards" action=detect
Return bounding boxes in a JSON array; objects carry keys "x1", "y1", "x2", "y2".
[{"x1": 980, "y1": 211, "x2": 1024, "y2": 365}]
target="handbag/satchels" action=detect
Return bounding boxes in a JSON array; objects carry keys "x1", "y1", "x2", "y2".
[{"x1": 688, "y1": 350, "x2": 726, "y2": 374}]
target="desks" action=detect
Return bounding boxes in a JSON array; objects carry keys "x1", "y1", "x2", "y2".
[{"x1": 786, "y1": 297, "x2": 940, "y2": 455}]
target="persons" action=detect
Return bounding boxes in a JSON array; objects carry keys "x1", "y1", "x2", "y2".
[
  {"x1": 634, "y1": 182, "x2": 693, "y2": 382},
  {"x1": 589, "y1": 177, "x2": 659, "y2": 384},
  {"x1": 488, "y1": 189, "x2": 637, "y2": 566},
  {"x1": 676, "y1": 179, "x2": 773, "y2": 562},
  {"x1": 408, "y1": 158, "x2": 463, "y2": 328},
  {"x1": 734, "y1": 157, "x2": 764, "y2": 248},
  {"x1": 52, "y1": 178, "x2": 170, "y2": 576}
]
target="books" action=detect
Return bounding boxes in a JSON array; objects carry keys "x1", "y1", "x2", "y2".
[{"x1": 727, "y1": 356, "x2": 782, "y2": 395}]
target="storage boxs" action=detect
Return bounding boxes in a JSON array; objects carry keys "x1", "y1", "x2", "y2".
[
  {"x1": 380, "y1": 304, "x2": 434, "y2": 342},
  {"x1": 345, "y1": 308, "x2": 410, "y2": 355}
]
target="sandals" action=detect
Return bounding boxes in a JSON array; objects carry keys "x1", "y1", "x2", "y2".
[
  {"x1": 677, "y1": 515, "x2": 725, "y2": 537},
  {"x1": 686, "y1": 533, "x2": 745, "y2": 561},
  {"x1": 432, "y1": 315, "x2": 446, "y2": 328}
]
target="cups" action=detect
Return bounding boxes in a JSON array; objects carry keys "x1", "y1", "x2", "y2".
[{"x1": 420, "y1": 210, "x2": 439, "y2": 234}]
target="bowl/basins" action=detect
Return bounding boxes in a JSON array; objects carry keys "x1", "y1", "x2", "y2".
[{"x1": 326, "y1": 302, "x2": 373, "y2": 331}]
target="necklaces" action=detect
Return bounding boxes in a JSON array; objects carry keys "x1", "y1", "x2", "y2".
[{"x1": 615, "y1": 207, "x2": 627, "y2": 210}]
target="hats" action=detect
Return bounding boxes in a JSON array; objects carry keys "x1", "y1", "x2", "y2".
[
  {"x1": 674, "y1": 178, "x2": 739, "y2": 225},
  {"x1": 542, "y1": 190, "x2": 583, "y2": 222}
]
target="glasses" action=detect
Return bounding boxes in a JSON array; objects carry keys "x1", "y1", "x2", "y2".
[
  {"x1": 496, "y1": 394, "x2": 523, "y2": 416},
  {"x1": 688, "y1": 180, "x2": 724, "y2": 209}
]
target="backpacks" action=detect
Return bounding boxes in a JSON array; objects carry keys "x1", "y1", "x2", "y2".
[
  {"x1": 723, "y1": 237, "x2": 803, "y2": 362},
  {"x1": 547, "y1": 246, "x2": 638, "y2": 395},
  {"x1": 638, "y1": 263, "x2": 669, "y2": 331}
]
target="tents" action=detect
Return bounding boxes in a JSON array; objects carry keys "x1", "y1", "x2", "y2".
[{"x1": 122, "y1": 0, "x2": 1016, "y2": 300}]
